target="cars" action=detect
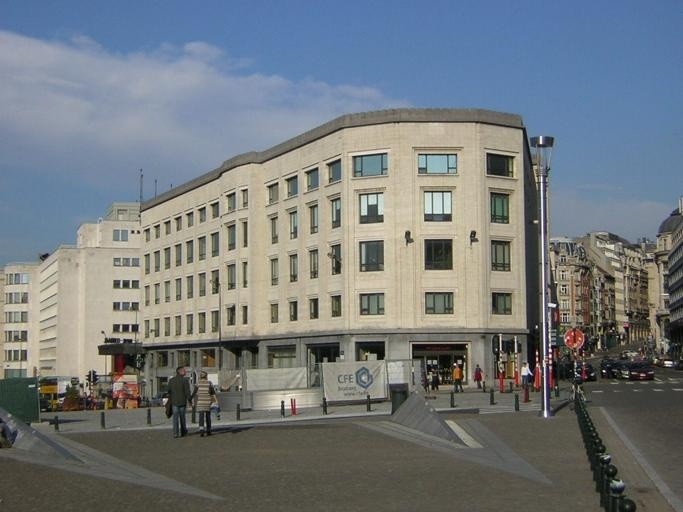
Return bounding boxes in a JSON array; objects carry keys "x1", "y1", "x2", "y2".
[
  {"x1": 559, "y1": 334, "x2": 682, "y2": 381},
  {"x1": 39, "y1": 393, "x2": 104, "y2": 412},
  {"x1": 147, "y1": 390, "x2": 168, "y2": 407}
]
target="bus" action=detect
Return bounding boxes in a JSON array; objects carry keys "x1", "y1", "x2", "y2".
[{"x1": 38, "y1": 374, "x2": 77, "y2": 406}]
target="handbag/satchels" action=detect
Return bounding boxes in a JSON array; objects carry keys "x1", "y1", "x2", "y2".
[{"x1": 165, "y1": 399, "x2": 173, "y2": 418}]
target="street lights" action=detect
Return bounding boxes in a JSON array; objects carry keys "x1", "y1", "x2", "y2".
[
  {"x1": 208, "y1": 279, "x2": 224, "y2": 372},
  {"x1": 17, "y1": 337, "x2": 23, "y2": 377},
  {"x1": 100, "y1": 329, "x2": 107, "y2": 382},
  {"x1": 526, "y1": 131, "x2": 556, "y2": 419},
  {"x1": 127, "y1": 306, "x2": 137, "y2": 373}
]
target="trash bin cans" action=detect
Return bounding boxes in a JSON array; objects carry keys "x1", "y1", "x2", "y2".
[{"x1": 391, "y1": 388, "x2": 407, "y2": 415}]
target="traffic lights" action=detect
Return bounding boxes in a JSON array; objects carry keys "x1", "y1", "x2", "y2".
[
  {"x1": 85, "y1": 371, "x2": 91, "y2": 382},
  {"x1": 92, "y1": 370, "x2": 98, "y2": 382}
]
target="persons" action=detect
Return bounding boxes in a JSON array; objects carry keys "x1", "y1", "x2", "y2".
[
  {"x1": 520, "y1": 362, "x2": 533, "y2": 390},
  {"x1": 472, "y1": 363, "x2": 484, "y2": 388},
  {"x1": 571, "y1": 367, "x2": 587, "y2": 401},
  {"x1": 188, "y1": 370, "x2": 219, "y2": 437},
  {"x1": 166, "y1": 366, "x2": 192, "y2": 439},
  {"x1": 452, "y1": 364, "x2": 463, "y2": 392},
  {"x1": 429, "y1": 367, "x2": 439, "y2": 390}
]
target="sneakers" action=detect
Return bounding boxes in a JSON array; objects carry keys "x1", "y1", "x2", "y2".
[{"x1": 173, "y1": 430, "x2": 211, "y2": 438}]
